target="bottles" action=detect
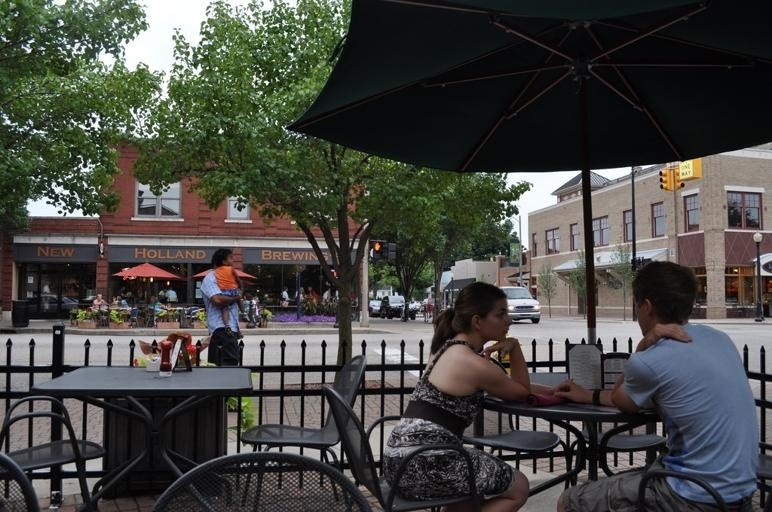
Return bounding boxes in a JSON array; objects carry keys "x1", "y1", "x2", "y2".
[{"x1": 159, "y1": 341, "x2": 173, "y2": 377}]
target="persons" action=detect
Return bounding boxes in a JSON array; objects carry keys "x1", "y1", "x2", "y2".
[
  {"x1": 421, "y1": 302, "x2": 427, "y2": 322},
  {"x1": 92, "y1": 285, "x2": 179, "y2": 311},
  {"x1": 427, "y1": 297, "x2": 433, "y2": 322},
  {"x1": 281, "y1": 280, "x2": 332, "y2": 305},
  {"x1": 381, "y1": 282, "x2": 575, "y2": 512},
  {"x1": 214, "y1": 249, "x2": 250, "y2": 335},
  {"x1": 556, "y1": 260, "x2": 759, "y2": 512},
  {"x1": 200, "y1": 253, "x2": 245, "y2": 412}
]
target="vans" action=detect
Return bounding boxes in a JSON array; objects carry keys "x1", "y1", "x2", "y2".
[{"x1": 498, "y1": 287, "x2": 541, "y2": 323}]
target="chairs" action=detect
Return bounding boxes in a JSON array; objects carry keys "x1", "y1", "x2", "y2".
[
  {"x1": 147, "y1": 447, "x2": 372, "y2": 512},
  {"x1": 0, "y1": 394, "x2": 109, "y2": 512},
  {"x1": 575, "y1": 351, "x2": 668, "y2": 472},
  {"x1": 236, "y1": 352, "x2": 370, "y2": 509},
  {"x1": 0, "y1": 447, "x2": 50, "y2": 512},
  {"x1": 318, "y1": 383, "x2": 491, "y2": 512},
  {"x1": 453, "y1": 392, "x2": 575, "y2": 494}
]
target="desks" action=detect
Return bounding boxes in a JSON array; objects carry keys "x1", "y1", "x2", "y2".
[
  {"x1": 480, "y1": 368, "x2": 667, "y2": 493},
  {"x1": 30, "y1": 361, "x2": 256, "y2": 508}
]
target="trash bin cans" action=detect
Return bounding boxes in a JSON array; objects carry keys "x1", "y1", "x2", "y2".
[
  {"x1": 12, "y1": 300, "x2": 30, "y2": 328},
  {"x1": 768, "y1": 302, "x2": 772, "y2": 317}
]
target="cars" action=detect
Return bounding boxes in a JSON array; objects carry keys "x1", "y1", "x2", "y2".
[{"x1": 369, "y1": 295, "x2": 421, "y2": 320}]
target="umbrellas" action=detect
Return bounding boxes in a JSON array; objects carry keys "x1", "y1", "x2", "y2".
[
  {"x1": 111, "y1": 262, "x2": 182, "y2": 299},
  {"x1": 286, "y1": 0, "x2": 772, "y2": 482},
  {"x1": 193, "y1": 268, "x2": 258, "y2": 280}
]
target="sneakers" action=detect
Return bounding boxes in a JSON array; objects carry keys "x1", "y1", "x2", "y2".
[
  {"x1": 242, "y1": 313, "x2": 250, "y2": 322},
  {"x1": 225, "y1": 326, "x2": 234, "y2": 337}
]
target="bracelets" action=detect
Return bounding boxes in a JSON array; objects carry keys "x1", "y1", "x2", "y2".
[{"x1": 592, "y1": 387, "x2": 602, "y2": 407}]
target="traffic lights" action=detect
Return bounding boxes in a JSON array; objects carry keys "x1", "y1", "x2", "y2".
[
  {"x1": 369, "y1": 241, "x2": 396, "y2": 261},
  {"x1": 659, "y1": 168, "x2": 685, "y2": 192}
]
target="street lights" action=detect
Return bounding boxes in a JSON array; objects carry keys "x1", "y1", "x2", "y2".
[{"x1": 753, "y1": 231, "x2": 764, "y2": 321}]
